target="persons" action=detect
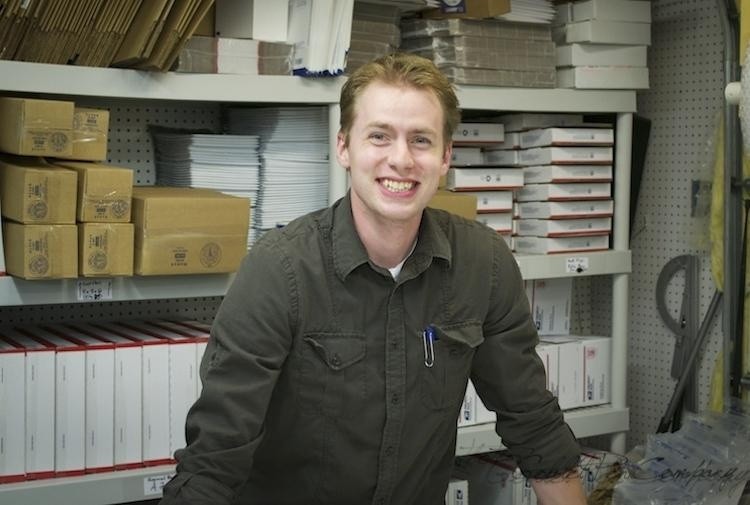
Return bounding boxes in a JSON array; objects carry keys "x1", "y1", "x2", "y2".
[{"x1": 154, "y1": 47, "x2": 588, "y2": 505}]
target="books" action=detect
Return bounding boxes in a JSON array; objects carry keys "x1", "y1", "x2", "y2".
[
  {"x1": 182, "y1": 0, "x2": 356, "y2": 74},
  {"x1": 154, "y1": 104, "x2": 331, "y2": 256}
]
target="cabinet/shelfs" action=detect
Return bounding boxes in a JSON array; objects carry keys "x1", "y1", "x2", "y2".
[{"x1": 0, "y1": 59, "x2": 634, "y2": 505}]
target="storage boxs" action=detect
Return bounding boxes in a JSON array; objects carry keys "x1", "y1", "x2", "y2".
[{"x1": 459, "y1": 278, "x2": 618, "y2": 428}]
